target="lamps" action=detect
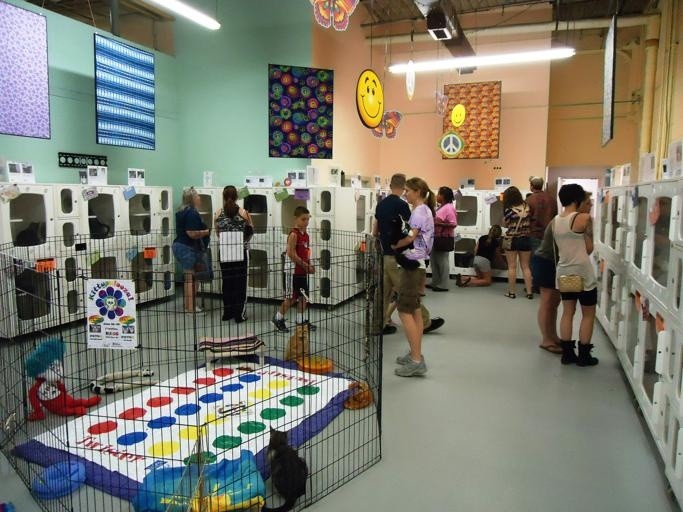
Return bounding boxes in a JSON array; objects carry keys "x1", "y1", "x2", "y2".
[
  {"x1": 376, "y1": 0, "x2": 577, "y2": 76},
  {"x1": 147, "y1": 0, "x2": 222, "y2": 34}
]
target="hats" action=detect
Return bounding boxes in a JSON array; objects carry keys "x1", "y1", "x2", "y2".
[{"x1": 528, "y1": 175, "x2": 544, "y2": 187}]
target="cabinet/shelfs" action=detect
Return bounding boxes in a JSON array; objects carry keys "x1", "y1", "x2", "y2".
[{"x1": 587, "y1": 171, "x2": 682, "y2": 510}]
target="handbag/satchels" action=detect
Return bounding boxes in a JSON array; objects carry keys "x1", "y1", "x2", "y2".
[
  {"x1": 193, "y1": 248, "x2": 214, "y2": 283},
  {"x1": 501, "y1": 235, "x2": 513, "y2": 250},
  {"x1": 432, "y1": 224, "x2": 454, "y2": 253},
  {"x1": 243, "y1": 225, "x2": 254, "y2": 241},
  {"x1": 556, "y1": 274, "x2": 585, "y2": 293}
]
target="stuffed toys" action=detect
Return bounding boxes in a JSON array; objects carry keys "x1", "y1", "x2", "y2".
[{"x1": 23, "y1": 338, "x2": 100, "y2": 422}]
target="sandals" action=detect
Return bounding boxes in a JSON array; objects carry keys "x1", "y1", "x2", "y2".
[
  {"x1": 526, "y1": 293, "x2": 533, "y2": 300},
  {"x1": 504, "y1": 291, "x2": 516, "y2": 298}
]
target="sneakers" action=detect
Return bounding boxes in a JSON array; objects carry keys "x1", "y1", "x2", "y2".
[
  {"x1": 182, "y1": 305, "x2": 207, "y2": 317},
  {"x1": 395, "y1": 354, "x2": 427, "y2": 377},
  {"x1": 244, "y1": 316, "x2": 248, "y2": 321},
  {"x1": 431, "y1": 287, "x2": 449, "y2": 292},
  {"x1": 295, "y1": 319, "x2": 317, "y2": 331},
  {"x1": 370, "y1": 324, "x2": 397, "y2": 337},
  {"x1": 269, "y1": 314, "x2": 289, "y2": 333},
  {"x1": 396, "y1": 350, "x2": 412, "y2": 366},
  {"x1": 422, "y1": 316, "x2": 445, "y2": 335},
  {"x1": 424, "y1": 284, "x2": 432, "y2": 289}
]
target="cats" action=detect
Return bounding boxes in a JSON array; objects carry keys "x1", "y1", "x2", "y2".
[
  {"x1": 261, "y1": 424, "x2": 308, "y2": 512},
  {"x1": 285, "y1": 324, "x2": 308, "y2": 363}
]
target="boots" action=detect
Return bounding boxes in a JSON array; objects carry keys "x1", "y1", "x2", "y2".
[
  {"x1": 560, "y1": 339, "x2": 578, "y2": 365},
  {"x1": 575, "y1": 341, "x2": 599, "y2": 367}
]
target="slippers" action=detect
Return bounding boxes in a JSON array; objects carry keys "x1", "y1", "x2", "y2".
[
  {"x1": 455, "y1": 273, "x2": 462, "y2": 286},
  {"x1": 537, "y1": 335, "x2": 563, "y2": 354},
  {"x1": 458, "y1": 277, "x2": 471, "y2": 288}
]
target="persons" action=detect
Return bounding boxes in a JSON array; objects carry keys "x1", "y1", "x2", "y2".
[
  {"x1": 550, "y1": 182, "x2": 599, "y2": 367},
  {"x1": 455, "y1": 224, "x2": 502, "y2": 287},
  {"x1": 423, "y1": 185, "x2": 456, "y2": 291},
  {"x1": 270, "y1": 207, "x2": 318, "y2": 332},
  {"x1": 530, "y1": 191, "x2": 595, "y2": 354},
  {"x1": 212, "y1": 185, "x2": 252, "y2": 323},
  {"x1": 370, "y1": 172, "x2": 445, "y2": 338},
  {"x1": 170, "y1": 186, "x2": 206, "y2": 314},
  {"x1": 387, "y1": 176, "x2": 435, "y2": 376},
  {"x1": 499, "y1": 185, "x2": 535, "y2": 300},
  {"x1": 523, "y1": 176, "x2": 557, "y2": 293}
]
